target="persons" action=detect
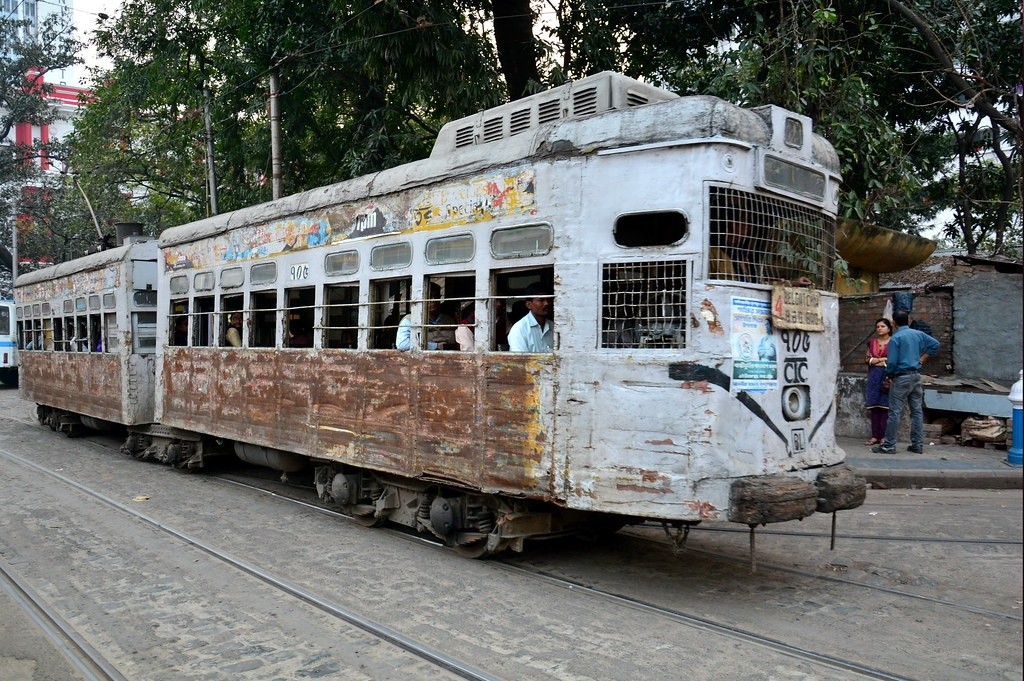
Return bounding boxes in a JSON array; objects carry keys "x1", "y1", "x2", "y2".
[
  {"x1": 96, "y1": 331, "x2": 102, "y2": 352},
  {"x1": 395, "y1": 281, "x2": 458, "y2": 350},
  {"x1": 872, "y1": 308, "x2": 940, "y2": 454},
  {"x1": 174, "y1": 316, "x2": 188, "y2": 346},
  {"x1": 26, "y1": 332, "x2": 41, "y2": 350},
  {"x1": 225, "y1": 313, "x2": 242, "y2": 346},
  {"x1": 603, "y1": 268, "x2": 684, "y2": 348},
  {"x1": 507, "y1": 282, "x2": 554, "y2": 349},
  {"x1": 864, "y1": 318, "x2": 894, "y2": 446},
  {"x1": 441, "y1": 284, "x2": 529, "y2": 351},
  {"x1": 70, "y1": 330, "x2": 88, "y2": 352},
  {"x1": 383, "y1": 315, "x2": 396, "y2": 348},
  {"x1": 290, "y1": 319, "x2": 310, "y2": 344},
  {"x1": 710, "y1": 222, "x2": 819, "y2": 286}
]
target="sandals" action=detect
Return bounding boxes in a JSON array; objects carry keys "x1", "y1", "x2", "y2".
[
  {"x1": 880, "y1": 438, "x2": 884, "y2": 445},
  {"x1": 864, "y1": 436, "x2": 878, "y2": 445}
]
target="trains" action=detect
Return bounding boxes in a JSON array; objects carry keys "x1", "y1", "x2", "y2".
[{"x1": 13, "y1": 70, "x2": 868, "y2": 560}]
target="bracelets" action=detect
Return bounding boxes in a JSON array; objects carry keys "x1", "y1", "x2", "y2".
[
  {"x1": 878, "y1": 358, "x2": 880, "y2": 362},
  {"x1": 921, "y1": 362, "x2": 924, "y2": 364}
]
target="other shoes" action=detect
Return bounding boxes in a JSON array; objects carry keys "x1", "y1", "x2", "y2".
[
  {"x1": 908, "y1": 446, "x2": 923, "y2": 454},
  {"x1": 873, "y1": 445, "x2": 897, "y2": 454}
]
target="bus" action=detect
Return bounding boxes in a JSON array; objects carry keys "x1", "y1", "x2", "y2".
[{"x1": 0, "y1": 299, "x2": 21, "y2": 388}]
